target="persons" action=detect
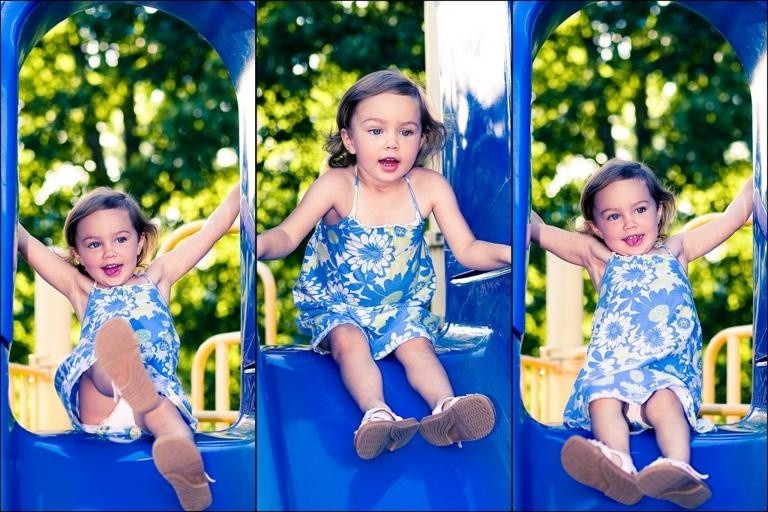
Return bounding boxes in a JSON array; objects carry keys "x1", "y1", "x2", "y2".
[
  {"x1": 531, "y1": 157, "x2": 754, "y2": 509},
  {"x1": 17, "y1": 183, "x2": 241, "y2": 511},
  {"x1": 256, "y1": 70, "x2": 512, "y2": 463}
]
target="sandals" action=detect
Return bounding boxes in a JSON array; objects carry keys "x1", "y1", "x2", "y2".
[
  {"x1": 561, "y1": 435, "x2": 644, "y2": 505},
  {"x1": 637, "y1": 457, "x2": 712, "y2": 508},
  {"x1": 419, "y1": 393, "x2": 496, "y2": 448},
  {"x1": 354, "y1": 408, "x2": 420, "y2": 459},
  {"x1": 96, "y1": 317, "x2": 159, "y2": 414},
  {"x1": 152, "y1": 434, "x2": 216, "y2": 512}
]
